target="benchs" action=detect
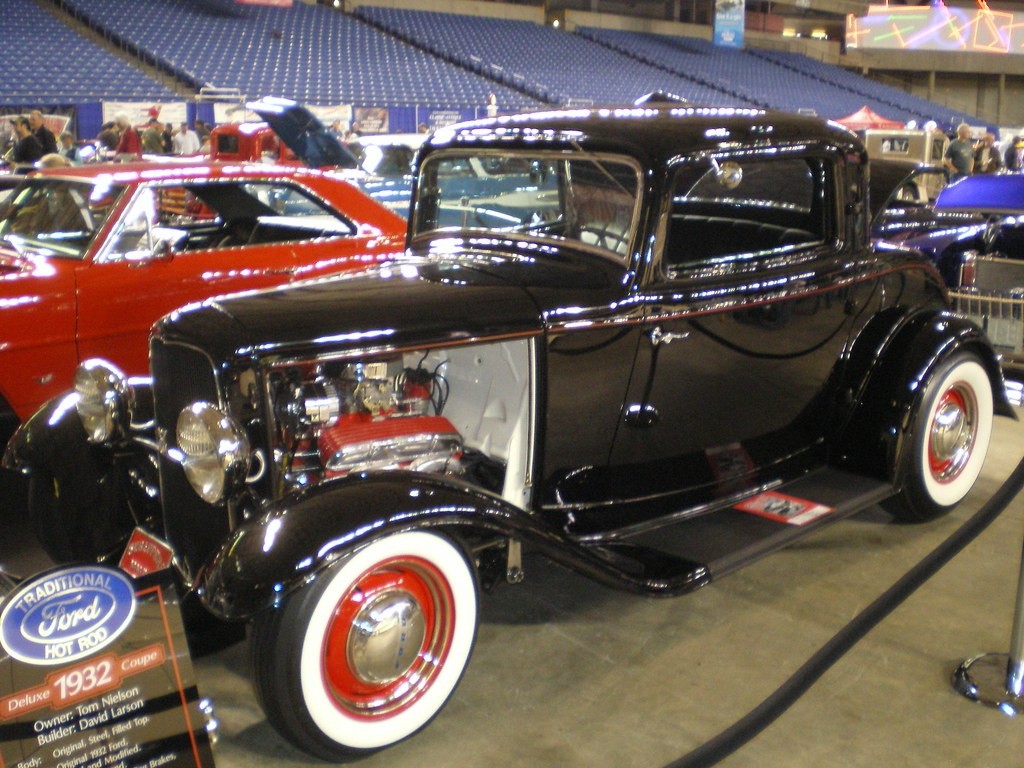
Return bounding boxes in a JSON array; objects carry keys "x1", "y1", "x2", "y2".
[{"x1": 579, "y1": 198, "x2": 821, "y2": 264}]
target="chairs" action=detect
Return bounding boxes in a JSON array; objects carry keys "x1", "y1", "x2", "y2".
[
  {"x1": 578, "y1": 201, "x2": 637, "y2": 255},
  {"x1": 210, "y1": 218, "x2": 264, "y2": 250}
]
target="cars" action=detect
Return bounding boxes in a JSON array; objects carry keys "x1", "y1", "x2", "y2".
[
  {"x1": 1, "y1": 101, "x2": 1019, "y2": 758},
  {"x1": 0, "y1": 163, "x2": 413, "y2": 483},
  {"x1": 863, "y1": 166, "x2": 1024, "y2": 288},
  {"x1": 287, "y1": 135, "x2": 563, "y2": 246}
]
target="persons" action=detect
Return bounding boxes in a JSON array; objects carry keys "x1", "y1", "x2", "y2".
[
  {"x1": 1, "y1": 110, "x2": 59, "y2": 175},
  {"x1": 944, "y1": 124, "x2": 974, "y2": 177},
  {"x1": 418, "y1": 123, "x2": 429, "y2": 134},
  {"x1": 96, "y1": 116, "x2": 142, "y2": 153},
  {"x1": 974, "y1": 133, "x2": 1001, "y2": 173},
  {"x1": 141, "y1": 118, "x2": 212, "y2": 154},
  {"x1": 59, "y1": 130, "x2": 81, "y2": 162},
  {"x1": 1005, "y1": 136, "x2": 1024, "y2": 171},
  {"x1": 351, "y1": 122, "x2": 362, "y2": 136},
  {"x1": 329, "y1": 119, "x2": 343, "y2": 137}
]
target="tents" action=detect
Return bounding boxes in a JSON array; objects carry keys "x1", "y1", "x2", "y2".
[{"x1": 834, "y1": 105, "x2": 904, "y2": 130}]
